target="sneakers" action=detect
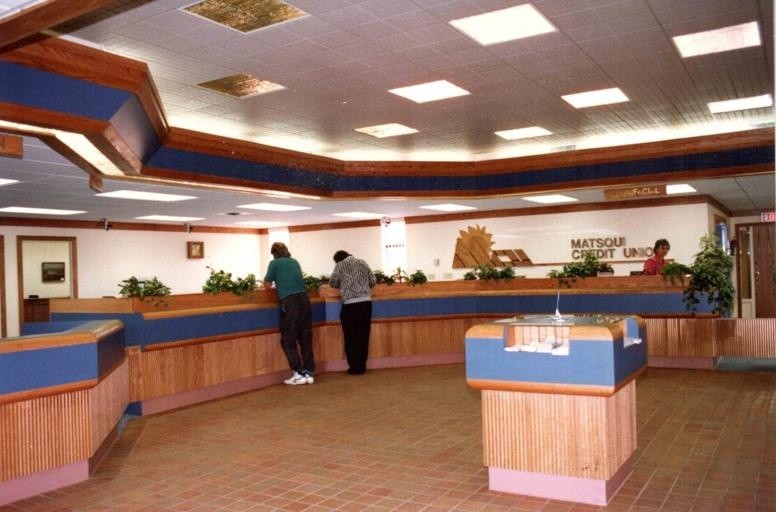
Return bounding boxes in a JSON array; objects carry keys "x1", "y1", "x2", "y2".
[{"x1": 283, "y1": 371, "x2": 314, "y2": 384}]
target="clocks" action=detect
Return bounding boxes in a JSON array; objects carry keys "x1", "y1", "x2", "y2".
[{"x1": 186, "y1": 240, "x2": 204, "y2": 259}]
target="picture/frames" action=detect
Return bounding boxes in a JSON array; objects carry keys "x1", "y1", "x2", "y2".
[{"x1": 41, "y1": 263, "x2": 65, "y2": 283}]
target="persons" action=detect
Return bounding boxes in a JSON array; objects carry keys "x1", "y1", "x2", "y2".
[
  {"x1": 643, "y1": 238, "x2": 677, "y2": 277},
  {"x1": 263, "y1": 241, "x2": 317, "y2": 387},
  {"x1": 329, "y1": 249, "x2": 376, "y2": 377}
]
